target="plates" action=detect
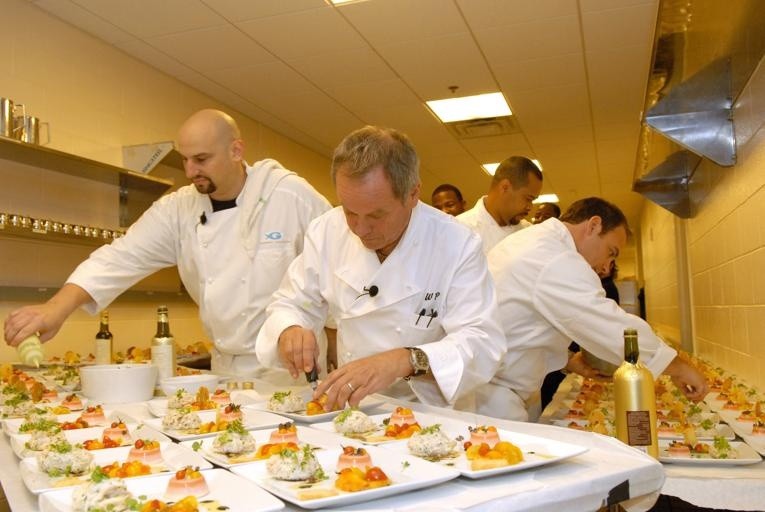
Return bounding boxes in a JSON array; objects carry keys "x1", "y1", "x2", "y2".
[
  {"x1": 550, "y1": 374, "x2": 765, "y2": 466},
  {"x1": 3, "y1": 386, "x2": 590, "y2": 512},
  {"x1": 0, "y1": 361, "x2": 96, "y2": 418}
]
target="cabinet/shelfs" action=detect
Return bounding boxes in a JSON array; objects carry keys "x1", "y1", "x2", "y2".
[{"x1": 0, "y1": 135, "x2": 181, "y2": 294}]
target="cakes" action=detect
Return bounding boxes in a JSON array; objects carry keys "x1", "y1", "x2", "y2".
[
  {"x1": 559, "y1": 341, "x2": 764, "y2": 460},
  {"x1": 103, "y1": 422, "x2": 131, "y2": 444},
  {"x1": 467, "y1": 424, "x2": 500, "y2": 449},
  {"x1": 81, "y1": 405, "x2": 105, "y2": 427},
  {"x1": 266, "y1": 423, "x2": 299, "y2": 447},
  {"x1": 211, "y1": 389, "x2": 231, "y2": 405},
  {"x1": 60, "y1": 394, "x2": 83, "y2": 411},
  {"x1": 128, "y1": 440, "x2": 163, "y2": 465},
  {"x1": 217, "y1": 403, "x2": 243, "y2": 425},
  {"x1": 3, "y1": 341, "x2": 213, "y2": 409},
  {"x1": 388, "y1": 406, "x2": 420, "y2": 432},
  {"x1": 336, "y1": 444, "x2": 372, "y2": 475},
  {"x1": 164, "y1": 465, "x2": 208, "y2": 501}
]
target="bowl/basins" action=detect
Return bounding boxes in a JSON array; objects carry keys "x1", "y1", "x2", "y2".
[
  {"x1": 159, "y1": 374, "x2": 230, "y2": 397},
  {"x1": 79, "y1": 363, "x2": 159, "y2": 401},
  {"x1": 581, "y1": 347, "x2": 618, "y2": 378}
]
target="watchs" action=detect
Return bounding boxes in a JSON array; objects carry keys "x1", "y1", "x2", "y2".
[{"x1": 407, "y1": 345, "x2": 430, "y2": 382}]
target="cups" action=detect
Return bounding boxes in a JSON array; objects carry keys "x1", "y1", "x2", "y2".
[
  {"x1": 0, "y1": 213, "x2": 127, "y2": 240},
  {"x1": 14, "y1": 115, "x2": 51, "y2": 146},
  {"x1": 0, "y1": 97, "x2": 27, "y2": 138}
]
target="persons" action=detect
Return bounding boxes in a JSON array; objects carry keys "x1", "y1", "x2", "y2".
[
  {"x1": 255, "y1": 126, "x2": 508, "y2": 413},
  {"x1": 540, "y1": 259, "x2": 620, "y2": 412},
  {"x1": 534, "y1": 202, "x2": 560, "y2": 223},
  {"x1": 4, "y1": 109, "x2": 337, "y2": 391},
  {"x1": 432, "y1": 184, "x2": 466, "y2": 219},
  {"x1": 471, "y1": 197, "x2": 711, "y2": 422},
  {"x1": 459, "y1": 157, "x2": 545, "y2": 256}
]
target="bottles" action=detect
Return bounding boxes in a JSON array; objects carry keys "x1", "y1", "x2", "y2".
[
  {"x1": 305, "y1": 357, "x2": 319, "y2": 392},
  {"x1": 613, "y1": 328, "x2": 660, "y2": 459},
  {"x1": 96, "y1": 309, "x2": 113, "y2": 365},
  {"x1": 16, "y1": 333, "x2": 42, "y2": 369},
  {"x1": 151, "y1": 304, "x2": 177, "y2": 392}
]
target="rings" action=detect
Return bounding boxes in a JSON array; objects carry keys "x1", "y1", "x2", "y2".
[{"x1": 348, "y1": 381, "x2": 355, "y2": 391}]
